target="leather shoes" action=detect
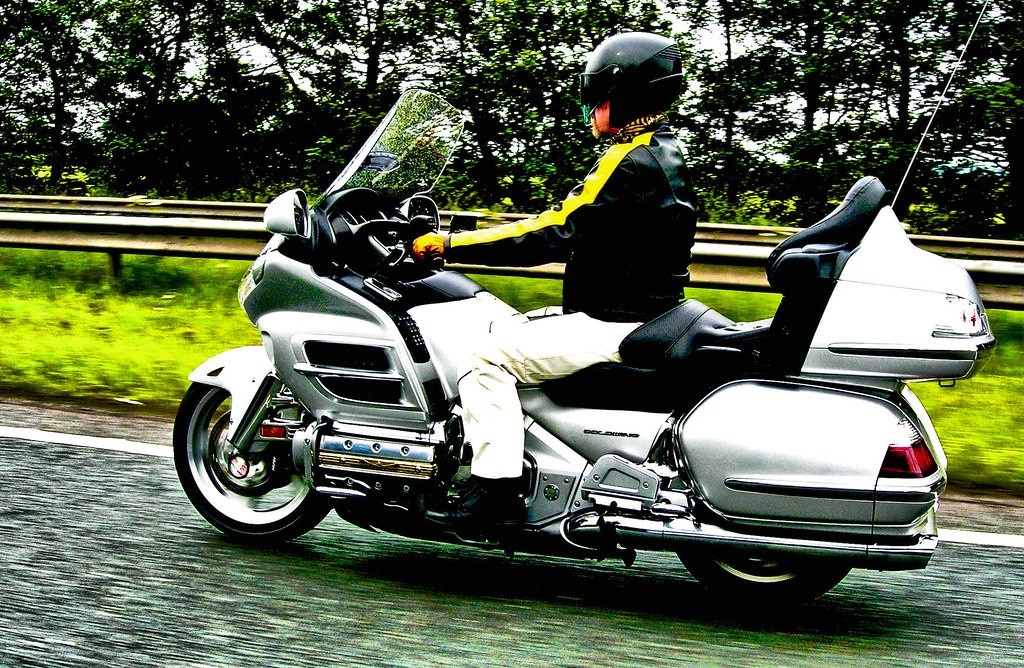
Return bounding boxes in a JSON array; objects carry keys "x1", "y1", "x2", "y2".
[{"x1": 420, "y1": 476, "x2": 528, "y2": 545}]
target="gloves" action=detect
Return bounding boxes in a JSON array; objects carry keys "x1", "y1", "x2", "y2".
[{"x1": 412, "y1": 231, "x2": 448, "y2": 261}]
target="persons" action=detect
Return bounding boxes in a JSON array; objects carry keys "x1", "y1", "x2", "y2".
[{"x1": 413, "y1": 32, "x2": 696, "y2": 533}]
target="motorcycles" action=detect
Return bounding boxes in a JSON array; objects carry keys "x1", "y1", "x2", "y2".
[{"x1": 171, "y1": 87, "x2": 999, "y2": 613}]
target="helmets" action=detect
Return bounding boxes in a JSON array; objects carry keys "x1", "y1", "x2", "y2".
[{"x1": 580, "y1": 32, "x2": 683, "y2": 128}]
